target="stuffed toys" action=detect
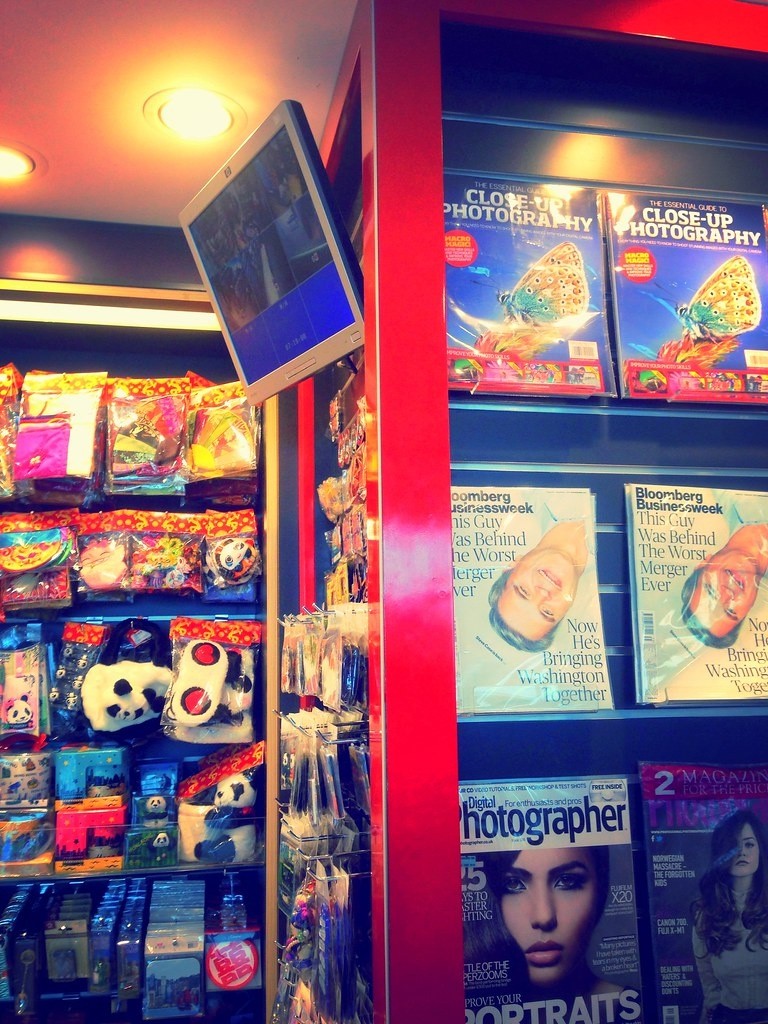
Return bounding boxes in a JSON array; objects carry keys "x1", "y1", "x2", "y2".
[{"x1": 194, "y1": 769, "x2": 257, "y2": 861}]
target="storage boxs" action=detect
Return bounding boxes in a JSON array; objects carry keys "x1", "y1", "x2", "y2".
[{"x1": 0, "y1": 745, "x2": 181, "y2": 877}]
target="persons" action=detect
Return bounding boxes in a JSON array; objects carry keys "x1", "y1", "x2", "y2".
[
  {"x1": 461, "y1": 845, "x2": 639, "y2": 1024},
  {"x1": 691, "y1": 809, "x2": 768, "y2": 1024},
  {"x1": 488, "y1": 489, "x2": 592, "y2": 655},
  {"x1": 680, "y1": 489, "x2": 768, "y2": 649}
]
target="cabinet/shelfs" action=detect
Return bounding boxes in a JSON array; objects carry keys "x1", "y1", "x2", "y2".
[{"x1": 0, "y1": 600, "x2": 266, "y2": 1024}]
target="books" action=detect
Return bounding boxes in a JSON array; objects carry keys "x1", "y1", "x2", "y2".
[
  {"x1": 443, "y1": 173, "x2": 768, "y2": 406},
  {"x1": 450, "y1": 484, "x2": 768, "y2": 713},
  {"x1": 456, "y1": 760, "x2": 768, "y2": 1024}
]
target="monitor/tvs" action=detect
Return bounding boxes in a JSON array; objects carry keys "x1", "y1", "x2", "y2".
[{"x1": 176, "y1": 100, "x2": 365, "y2": 406}]
